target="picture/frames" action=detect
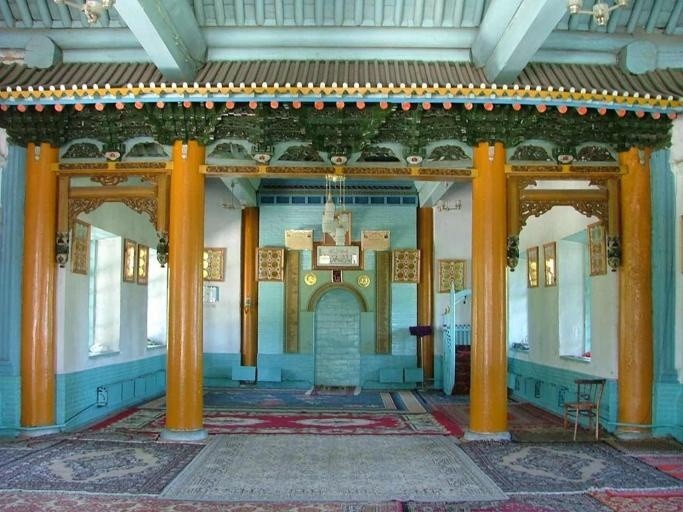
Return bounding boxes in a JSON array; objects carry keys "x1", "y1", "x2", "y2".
[
  {"x1": 71, "y1": 219, "x2": 91, "y2": 275},
  {"x1": 201, "y1": 246, "x2": 225, "y2": 281},
  {"x1": 123, "y1": 238, "x2": 151, "y2": 287},
  {"x1": 254, "y1": 209, "x2": 466, "y2": 294},
  {"x1": 526, "y1": 240, "x2": 558, "y2": 289},
  {"x1": 588, "y1": 220, "x2": 608, "y2": 279}
]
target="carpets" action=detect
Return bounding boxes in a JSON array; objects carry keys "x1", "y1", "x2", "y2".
[
  {"x1": 606, "y1": 432, "x2": 683, "y2": 481},
  {"x1": 455, "y1": 434, "x2": 678, "y2": 497},
  {"x1": 1, "y1": 432, "x2": 205, "y2": 496},
  {"x1": 1, "y1": 480, "x2": 683, "y2": 510},
  {"x1": 2, "y1": 446, "x2": 34, "y2": 466},
  {"x1": 160, "y1": 436, "x2": 509, "y2": 502},
  {"x1": 429, "y1": 402, "x2": 580, "y2": 435},
  {"x1": 86, "y1": 407, "x2": 463, "y2": 436},
  {"x1": 417, "y1": 386, "x2": 524, "y2": 407},
  {"x1": 137, "y1": 387, "x2": 427, "y2": 413}
]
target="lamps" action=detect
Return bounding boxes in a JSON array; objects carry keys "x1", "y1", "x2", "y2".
[
  {"x1": 567, "y1": 0, "x2": 628, "y2": 27},
  {"x1": 54, "y1": 0, "x2": 116, "y2": 23},
  {"x1": 321, "y1": 172, "x2": 350, "y2": 245}
]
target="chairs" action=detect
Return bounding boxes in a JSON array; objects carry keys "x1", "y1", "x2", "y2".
[{"x1": 562, "y1": 377, "x2": 609, "y2": 441}]
